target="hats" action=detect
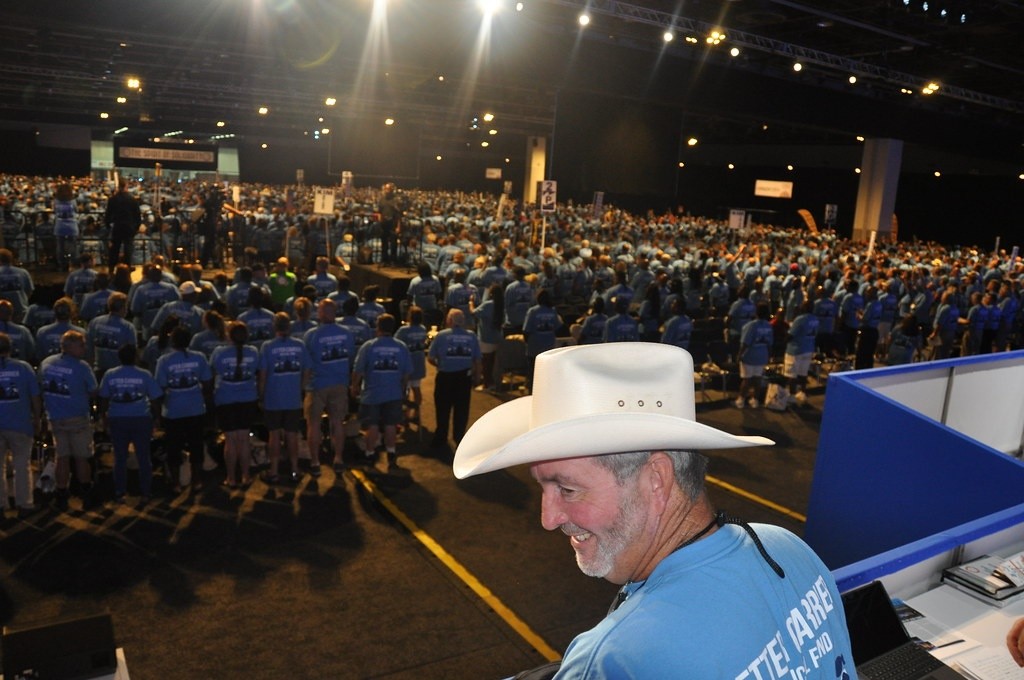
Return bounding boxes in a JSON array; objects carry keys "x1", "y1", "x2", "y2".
[{"x1": 452, "y1": 342, "x2": 777, "y2": 480}]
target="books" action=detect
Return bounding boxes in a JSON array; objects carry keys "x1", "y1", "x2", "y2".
[{"x1": 940, "y1": 555, "x2": 1024, "y2": 608}]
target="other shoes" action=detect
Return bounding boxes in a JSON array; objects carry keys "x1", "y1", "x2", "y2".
[
  {"x1": 0, "y1": 447, "x2": 398, "y2": 520},
  {"x1": 733, "y1": 396, "x2": 744, "y2": 408},
  {"x1": 748, "y1": 396, "x2": 759, "y2": 408}
]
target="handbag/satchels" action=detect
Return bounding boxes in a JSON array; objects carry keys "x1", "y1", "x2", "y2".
[{"x1": 764, "y1": 382, "x2": 791, "y2": 411}]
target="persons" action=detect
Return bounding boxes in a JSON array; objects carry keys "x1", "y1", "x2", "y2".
[
  {"x1": 0, "y1": 174, "x2": 1024, "y2": 512},
  {"x1": 451, "y1": 342, "x2": 856, "y2": 680}
]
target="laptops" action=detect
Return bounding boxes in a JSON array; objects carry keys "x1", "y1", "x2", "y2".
[{"x1": 841, "y1": 581, "x2": 969, "y2": 680}]
